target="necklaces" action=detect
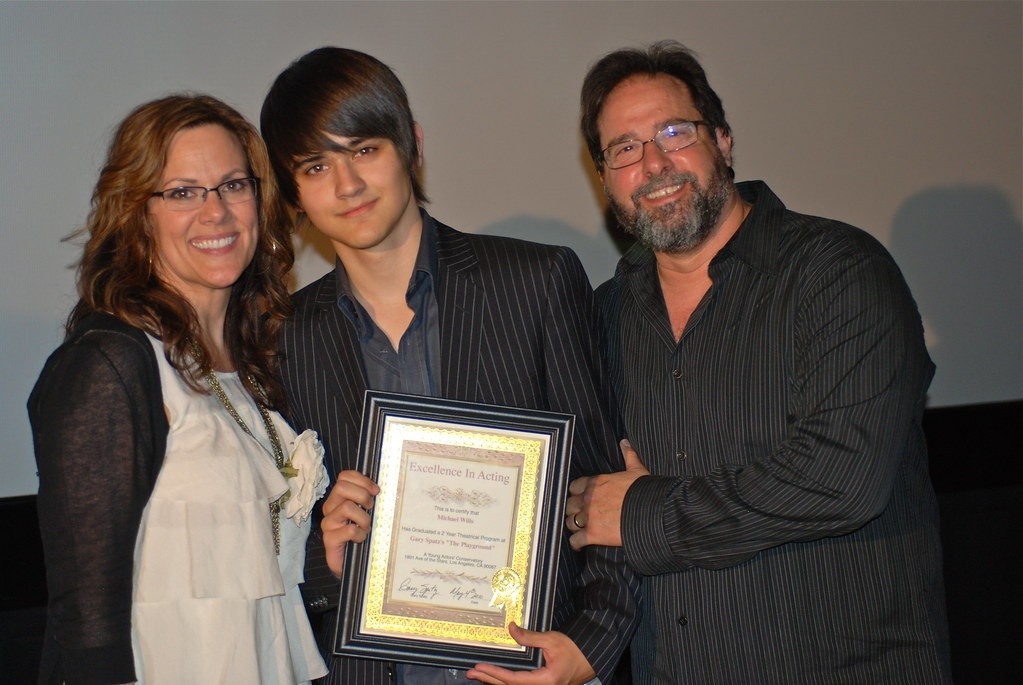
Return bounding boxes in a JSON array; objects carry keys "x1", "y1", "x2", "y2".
[{"x1": 190, "y1": 339, "x2": 285, "y2": 555}]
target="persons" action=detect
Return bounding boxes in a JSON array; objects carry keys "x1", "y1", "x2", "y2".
[
  {"x1": 566, "y1": 40, "x2": 953, "y2": 685},
  {"x1": 27, "y1": 95, "x2": 329, "y2": 685},
  {"x1": 250, "y1": 46, "x2": 645, "y2": 685}
]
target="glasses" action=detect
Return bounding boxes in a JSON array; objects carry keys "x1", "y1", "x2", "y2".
[
  {"x1": 150, "y1": 178, "x2": 257, "y2": 211},
  {"x1": 597, "y1": 121, "x2": 708, "y2": 170}
]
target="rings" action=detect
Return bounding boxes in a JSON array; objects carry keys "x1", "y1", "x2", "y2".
[{"x1": 574, "y1": 514, "x2": 584, "y2": 528}]
